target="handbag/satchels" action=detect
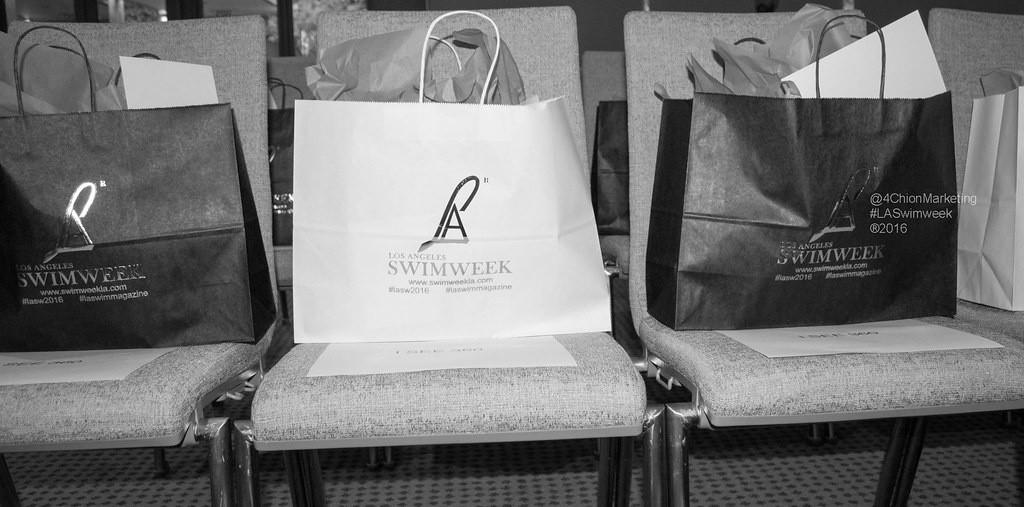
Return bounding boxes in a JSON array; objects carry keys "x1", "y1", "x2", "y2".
[
  {"x1": 591, "y1": 100, "x2": 634, "y2": 277},
  {"x1": 267, "y1": 77, "x2": 302, "y2": 247},
  {"x1": 0, "y1": 24, "x2": 276, "y2": 354},
  {"x1": 292, "y1": 9, "x2": 612, "y2": 344},
  {"x1": 646, "y1": 15, "x2": 956, "y2": 332},
  {"x1": 957, "y1": 68, "x2": 1024, "y2": 310}
]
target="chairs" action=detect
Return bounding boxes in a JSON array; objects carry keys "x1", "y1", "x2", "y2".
[{"x1": 0, "y1": 0, "x2": 1024, "y2": 507}]
